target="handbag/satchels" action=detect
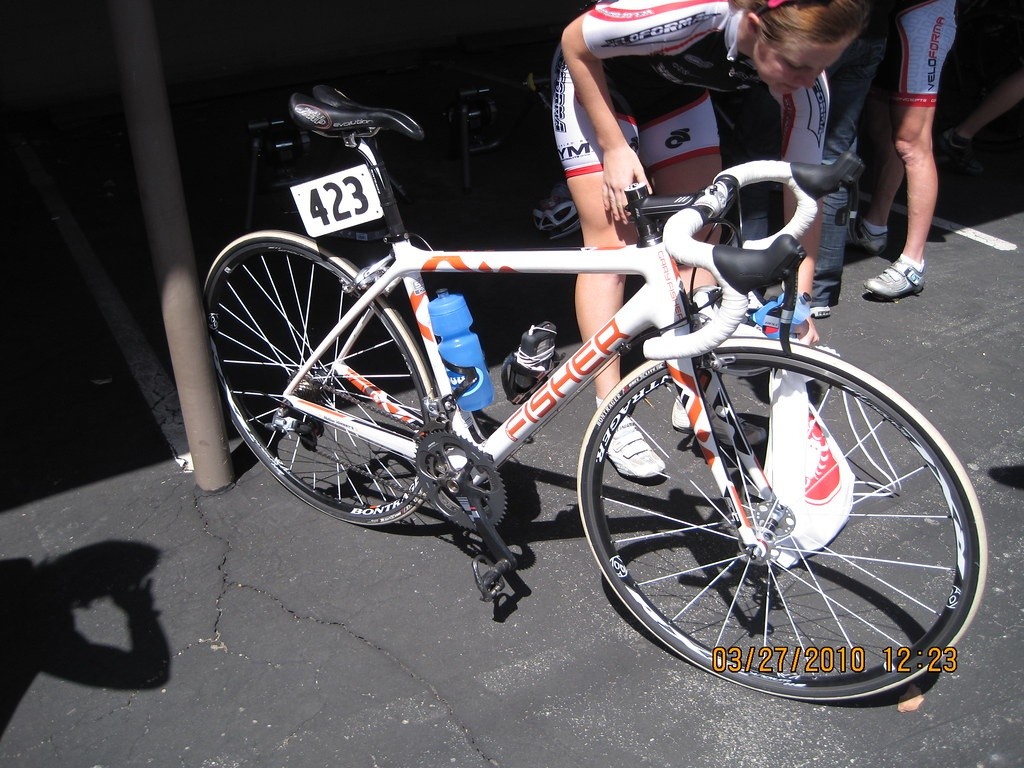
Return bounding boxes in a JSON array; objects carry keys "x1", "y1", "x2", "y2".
[{"x1": 753, "y1": 336, "x2": 856, "y2": 571}]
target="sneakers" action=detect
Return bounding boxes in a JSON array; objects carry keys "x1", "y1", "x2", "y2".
[
  {"x1": 671, "y1": 395, "x2": 767, "y2": 447},
  {"x1": 863, "y1": 259, "x2": 927, "y2": 302},
  {"x1": 606, "y1": 416, "x2": 666, "y2": 479},
  {"x1": 846, "y1": 222, "x2": 888, "y2": 256}
]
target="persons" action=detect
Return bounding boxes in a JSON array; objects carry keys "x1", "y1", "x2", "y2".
[
  {"x1": 541, "y1": 1, "x2": 874, "y2": 484},
  {"x1": 805, "y1": 0, "x2": 893, "y2": 321},
  {"x1": 844, "y1": 0, "x2": 961, "y2": 299},
  {"x1": 936, "y1": 63, "x2": 1024, "y2": 165}
]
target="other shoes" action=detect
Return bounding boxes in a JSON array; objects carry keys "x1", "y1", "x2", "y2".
[{"x1": 809, "y1": 299, "x2": 831, "y2": 319}]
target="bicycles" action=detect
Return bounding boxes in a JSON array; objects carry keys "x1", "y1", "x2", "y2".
[{"x1": 200, "y1": 85, "x2": 990, "y2": 710}]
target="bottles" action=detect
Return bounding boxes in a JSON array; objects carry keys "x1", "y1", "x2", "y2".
[
  {"x1": 751, "y1": 291, "x2": 812, "y2": 339},
  {"x1": 427, "y1": 288, "x2": 494, "y2": 412}
]
